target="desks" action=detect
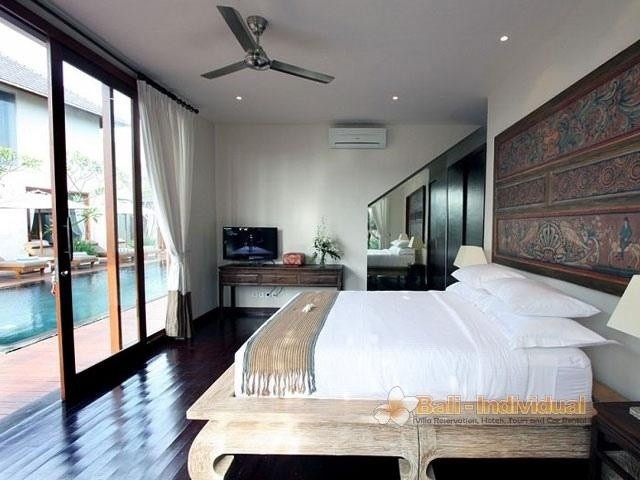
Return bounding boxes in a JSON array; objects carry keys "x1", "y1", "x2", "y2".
[{"x1": 219, "y1": 264, "x2": 344, "y2": 313}]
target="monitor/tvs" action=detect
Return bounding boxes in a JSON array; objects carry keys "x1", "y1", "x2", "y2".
[{"x1": 223, "y1": 227, "x2": 277, "y2": 267}]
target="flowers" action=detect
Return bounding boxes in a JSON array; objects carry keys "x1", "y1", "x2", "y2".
[{"x1": 308, "y1": 217, "x2": 343, "y2": 265}]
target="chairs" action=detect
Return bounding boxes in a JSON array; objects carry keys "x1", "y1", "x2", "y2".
[
  {"x1": 118, "y1": 239, "x2": 158, "y2": 262},
  {"x1": 0, "y1": 239, "x2": 98, "y2": 281}
]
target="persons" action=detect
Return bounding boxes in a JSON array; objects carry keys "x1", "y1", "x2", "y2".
[{"x1": 619, "y1": 216, "x2": 632, "y2": 258}]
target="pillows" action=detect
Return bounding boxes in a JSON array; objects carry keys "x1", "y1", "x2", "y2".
[
  {"x1": 388, "y1": 240, "x2": 413, "y2": 255},
  {"x1": 445, "y1": 265, "x2": 623, "y2": 350}
]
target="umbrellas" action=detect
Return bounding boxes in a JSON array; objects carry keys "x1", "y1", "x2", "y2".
[{"x1": 1, "y1": 189, "x2": 90, "y2": 257}]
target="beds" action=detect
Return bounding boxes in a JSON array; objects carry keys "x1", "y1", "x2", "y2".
[
  {"x1": 186, "y1": 291, "x2": 628, "y2": 479},
  {"x1": 366, "y1": 249, "x2": 422, "y2": 277}
]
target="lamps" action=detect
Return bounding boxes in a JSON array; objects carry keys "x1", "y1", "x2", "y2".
[
  {"x1": 452, "y1": 245, "x2": 488, "y2": 268},
  {"x1": 408, "y1": 236, "x2": 425, "y2": 264},
  {"x1": 398, "y1": 233, "x2": 408, "y2": 240},
  {"x1": 606, "y1": 275, "x2": 640, "y2": 421}
]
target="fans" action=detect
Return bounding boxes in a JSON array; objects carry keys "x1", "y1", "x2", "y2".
[{"x1": 199, "y1": 5, "x2": 337, "y2": 83}]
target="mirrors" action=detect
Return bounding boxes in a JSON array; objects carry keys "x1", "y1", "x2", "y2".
[{"x1": 366, "y1": 166, "x2": 429, "y2": 290}]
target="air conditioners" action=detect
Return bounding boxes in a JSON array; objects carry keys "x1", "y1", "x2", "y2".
[{"x1": 329, "y1": 126, "x2": 386, "y2": 151}]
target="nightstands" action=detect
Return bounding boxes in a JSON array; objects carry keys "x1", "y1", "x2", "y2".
[{"x1": 591, "y1": 400, "x2": 639, "y2": 479}]
